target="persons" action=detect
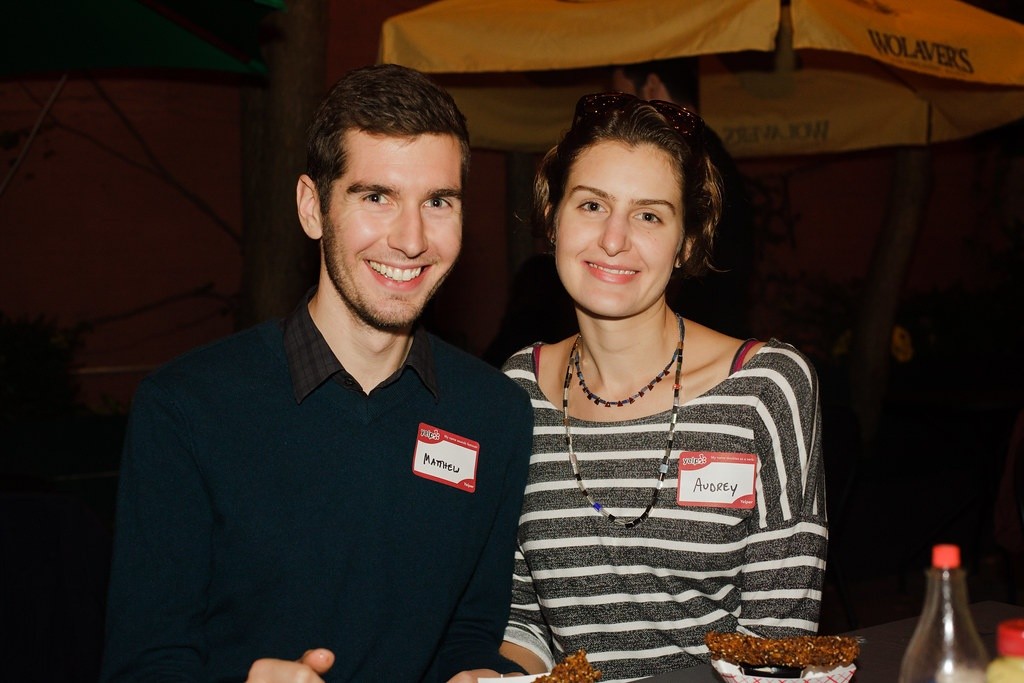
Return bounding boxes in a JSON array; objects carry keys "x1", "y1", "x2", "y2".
[
  {"x1": 497, "y1": 55, "x2": 830, "y2": 683},
  {"x1": 102, "y1": 64, "x2": 535, "y2": 682}
]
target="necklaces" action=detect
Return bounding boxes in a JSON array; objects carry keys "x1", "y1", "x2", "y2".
[{"x1": 561, "y1": 313, "x2": 685, "y2": 529}]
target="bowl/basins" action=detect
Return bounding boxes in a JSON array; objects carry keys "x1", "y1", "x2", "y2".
[{"x1": 711, "y1": 659, "x2": 856, "y2": 682}]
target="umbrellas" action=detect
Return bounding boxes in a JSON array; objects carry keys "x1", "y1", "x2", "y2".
[{"x1": 376, "y1": 0, "x2": 1004, "y2": 157}]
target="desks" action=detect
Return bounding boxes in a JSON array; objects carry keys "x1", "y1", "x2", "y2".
[{"x1": 627, "y1": 599, "x2": 1024, "y2": 682}]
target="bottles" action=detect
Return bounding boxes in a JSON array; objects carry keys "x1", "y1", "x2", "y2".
[
  {"x1": 898, "y1": 542, "x2": 989, "y2": 683},
  {"x1": 986, "y1": 615, "x2": 1024, "y2": 682}
]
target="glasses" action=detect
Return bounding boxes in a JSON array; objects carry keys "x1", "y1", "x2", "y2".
[{"x1": 571, "y1": 91, "x2": 701, "y2": 144}]
